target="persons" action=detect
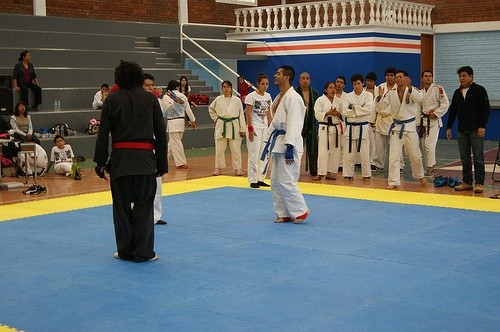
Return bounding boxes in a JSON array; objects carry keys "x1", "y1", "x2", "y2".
[
  {"x1": 12, "y1": 50, "x2": 42, "y2": 112},
  {"x1": 162, "y1": 79, "x2": 196, "y2": 169},
  {"x1": 416, "y1": 69, "x2": 449, "y2": 173},
  {"x1": 10, "y1": 102, "x2": 40, "y2": 146},
  {"x1": 446, "y1": 65, "x2": 490, "y2": 193},
  {"x1": 364, "y1": 68, "x2": 404, "y2": 175},
  {"x1": 93, "y1": 62, "x2": 169, "y2": 264},
  {"x1": 267, "y1": 66, "x2": 312, "y2": 224},
  {"x1": 244, "y1": 72, "x2": 272, "y2": 189},
  {"x1": 208, "y1": 80, "x2": 246, "y2": 175},
  {"x1": 143, "y1": 73, "x2": 186, "y2": 225},
  {"x1": 13, "y1": 135, "x2": 82, "y2": 179},
  {"x1": 180, "y1": 76, "x2": 192, "y2": 124},
  {"x1": 91, "y1": 84, "x2": 109, "y2": 110},
  {"x1": 375, "y1": 71, "x2": 428, "y2": 191},
  {"x1": 312, "y1": 77, "x2": 347, "y2": 181},
  {"x1": 294, "y1": 72, "x2": 320, "y2": 180},
  {"x1": 343, "y1": 73, "x2": 375, "y2": 183},
  {"x1": 238, "y1": 76, "x2": 253, "y2": 110}
]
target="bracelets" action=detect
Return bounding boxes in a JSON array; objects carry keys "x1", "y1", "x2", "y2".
[{"x1": 248, "y1": 126, "x2": 253, "y2": 132}]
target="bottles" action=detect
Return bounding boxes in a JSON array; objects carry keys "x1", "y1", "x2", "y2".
[
  {"x1": 54, "y1": 100, "x2": 57, "y2": 112},
  {"x1": 55, "y1": 126, "x2": 59, "y2": 135},
  {"x1": 61, "y1": 125, "x2": 64, "y2": 137},
  {"x1": 57, "y1": 100, "x2": 61, "y2": 111}
]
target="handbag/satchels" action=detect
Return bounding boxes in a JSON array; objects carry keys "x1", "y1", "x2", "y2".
[{"x1": 190, "y1": 94, "x2": 209, "y2": 105}]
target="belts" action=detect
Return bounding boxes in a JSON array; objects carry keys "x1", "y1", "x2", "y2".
[{"x1": 112, "y1": 142, "x2": 154, "y2": 150}]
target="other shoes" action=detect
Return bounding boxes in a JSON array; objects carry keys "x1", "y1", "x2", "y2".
[
  {"x1": 177, "y1": 165, "x2": 188, "y2": 169},
  {"x1": 71, "y1": 163, "x2": 82, "y2": 178},
  {"x1": 454, "y1": 183, "x2": 473, "y2": 191},
  {"x1": 343, "y1": 176, "x2": 354, "y2": 182},
  {"x1": 386, "y1": 185, "x2": 397, "y2": 189},
  {"x1": 474, "y1": 183, "x2": 484, "y2": 193},
  {"x1": 212, "y1": 168, "x2": 224, "y2": 176},
  {"x1": 22, "y1": 184, "x2": 47, "y2": 195},
  {"x1": 312, "y1": 175, "x2": 321, "y2": 181},
  {"x1": 234, "y1": 169, "x2": 244, "y2": 176},
  {"x1": 420, "y1": 178, "x2": 427, "y2": 184},
  {"x1": 325, "y1": 172, "x2": 336, "y2": 180},
  {"x1": 363, "y1": 177, "x2": 371, "y2": 184},
  {"x1": 26, "y1": 106, "x2": 39, "y2": 112}
]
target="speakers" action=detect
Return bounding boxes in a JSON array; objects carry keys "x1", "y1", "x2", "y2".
[{"x1": 0, "y1": 74, "x2": 14, "y2": 115}]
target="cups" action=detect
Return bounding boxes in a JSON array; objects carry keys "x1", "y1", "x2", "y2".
[{"x1": 10, "y1": 130, "x2": 14, "y2": 140}]
